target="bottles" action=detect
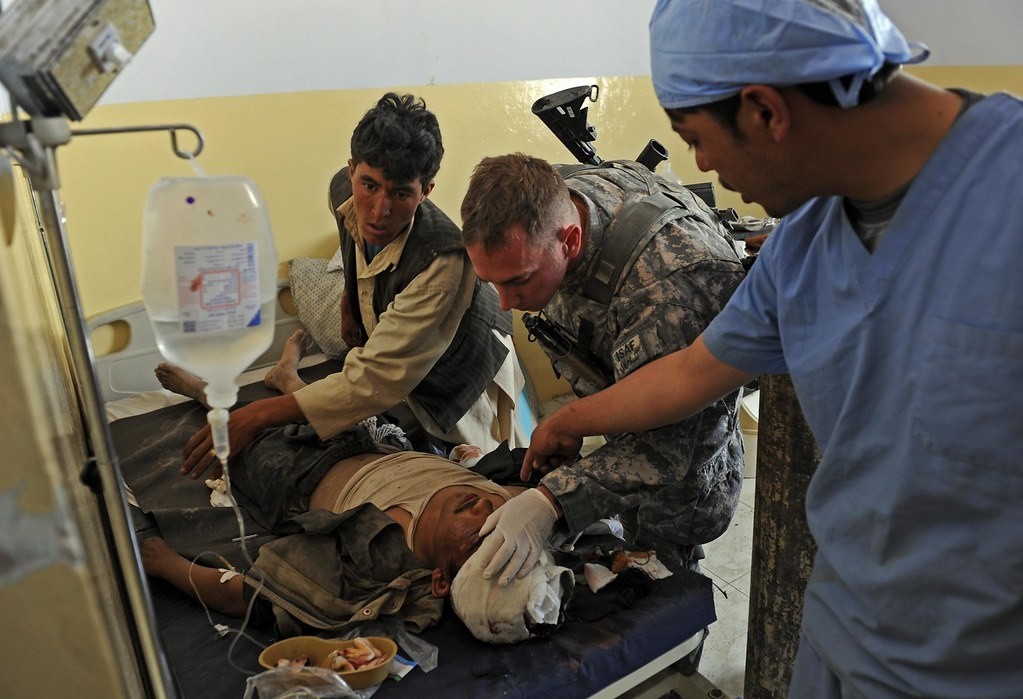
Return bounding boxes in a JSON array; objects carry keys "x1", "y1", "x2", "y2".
[{"x1": 142, "y1": 176, "x2": 275, "y2": 409}]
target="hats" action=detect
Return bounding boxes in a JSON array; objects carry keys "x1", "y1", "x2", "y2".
[{"x1": 649, "y1": 1, "x2": 932, "y2": 110}]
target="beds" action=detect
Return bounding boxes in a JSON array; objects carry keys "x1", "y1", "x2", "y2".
[{"x1": 84, "y1": 277, "x2": 718, "y2": 699}]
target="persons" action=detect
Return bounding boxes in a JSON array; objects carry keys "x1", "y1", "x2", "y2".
[
  {"x1": 460, "y1": 153, "x2": 744, "y2": 586},
  {"x1": 518, "y1": 1, "x2": 1023, "y2": 699},
  {"x1": 139, "y1": 328, "x2": 598, "y2": 645},
  {"x1": 179, "y1": 91, "x2": 526, "y2": 482}
]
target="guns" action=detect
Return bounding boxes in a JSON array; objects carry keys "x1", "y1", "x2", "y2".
[
  {"x1": 531, "y1": 83, "x2": 740, "y2": 233},
  {"x1": 522, "y1": 307, "x2": 616, "y2": 390}
]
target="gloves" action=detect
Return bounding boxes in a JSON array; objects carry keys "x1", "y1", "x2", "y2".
[{"x1": 479, "y1": 488, "x2": 558, "y2": 587}]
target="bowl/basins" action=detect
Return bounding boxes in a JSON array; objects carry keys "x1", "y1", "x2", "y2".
[{"x1": 258, "y1": 635, "x2": 397, "y2": 689}]
